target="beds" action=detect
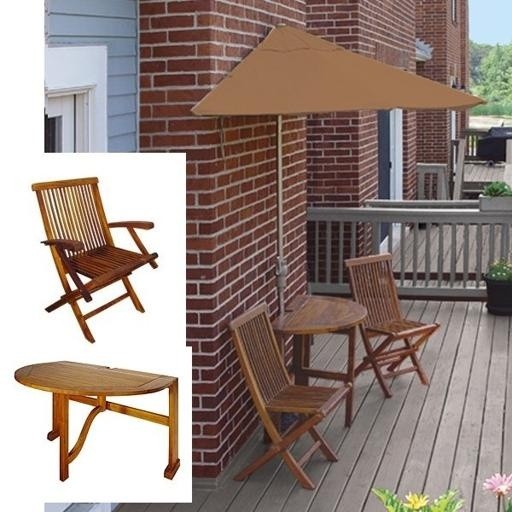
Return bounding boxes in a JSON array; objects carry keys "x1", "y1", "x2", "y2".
[{"x1": 13, "y1": 363, "x2": 181, "y2": 481}]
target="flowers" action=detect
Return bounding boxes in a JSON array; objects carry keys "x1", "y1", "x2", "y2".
[
  {"x1": 486, "y1": 256, "x2": 512, "y2": 280},
  {"x1": 483, "y1": 181, "x2": 512, "y2": 196}
]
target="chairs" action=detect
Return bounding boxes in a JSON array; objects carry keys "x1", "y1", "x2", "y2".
[
  {"x1": 229, "y1": 299, "x2": 352, "y2": 490},
  {"x1": 32, "y1": 177, "x2": 160, "y2": 344},
  {"x1": 343, "y1": 252, "x2": 440, "y2": 397}
]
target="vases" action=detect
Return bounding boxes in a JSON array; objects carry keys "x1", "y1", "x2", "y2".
[
  {"x1": 478, "y1": 194, "x2": 512, "y2": 212},
  {"x1": 483, "y1": 278, "x2": 512, "y2": 316}
]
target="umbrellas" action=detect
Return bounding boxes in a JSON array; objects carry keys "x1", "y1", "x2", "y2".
[{"x1": 191, "y1": 22, "x2": 487, "y2": 317}]
